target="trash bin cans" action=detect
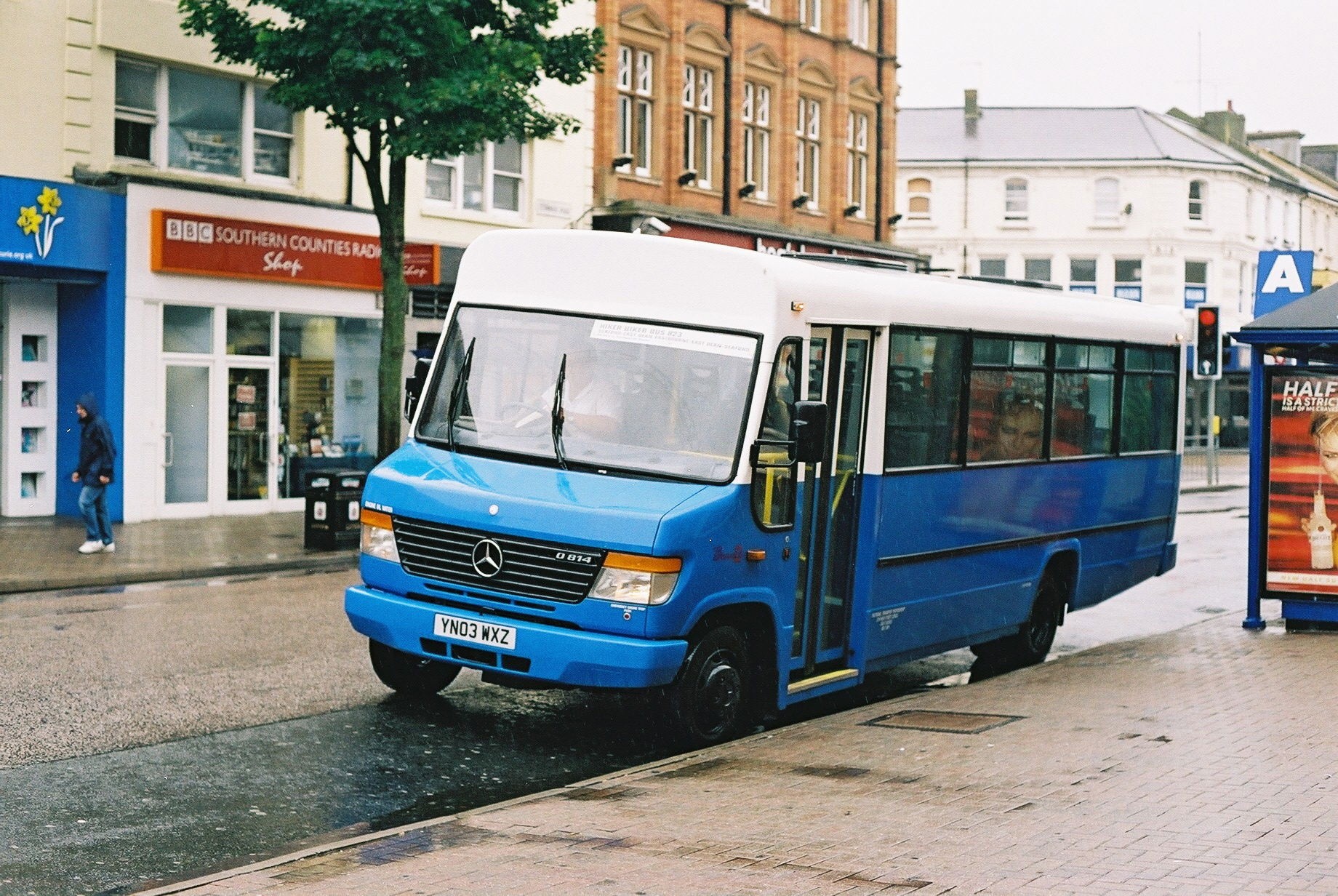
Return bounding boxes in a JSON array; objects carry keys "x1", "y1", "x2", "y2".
[{"x1": 304, "y1": 467, "x2": 368, "y2": 551}]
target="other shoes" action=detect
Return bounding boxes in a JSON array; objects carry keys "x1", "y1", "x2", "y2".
[
  {"x1": 78, "y1": 539, "x2": 103, "y2": 554},
  {"x1": 99, "y1": 542, "x2": 116, "y2": 553}
]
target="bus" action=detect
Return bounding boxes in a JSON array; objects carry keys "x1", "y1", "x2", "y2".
[{"x1": 344, "y1": 229, "x2": 1187, "y2": 745}]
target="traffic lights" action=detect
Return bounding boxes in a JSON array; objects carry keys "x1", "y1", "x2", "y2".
[{"x1": 1193, "y1": 302, "x2": 1223, "y2": 380}]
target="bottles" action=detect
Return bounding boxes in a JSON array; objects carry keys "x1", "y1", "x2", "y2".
[{"x1": 1309, "y1": 489, "x2": 1334, "y2": 570}]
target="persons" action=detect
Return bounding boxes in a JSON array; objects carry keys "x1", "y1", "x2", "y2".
[
  {"x1": 71, "y1": 394, "x2": 117, "y2": 554},
  {"x1": 1302, "y1": 401, "x2": 1338, "y2": 569},
  {"x1": 984, "y1": 392, "x2": 1047, "y2": 460},
  {"x1": 504, "y1": 340, "x2": 624, "y2": 444}
]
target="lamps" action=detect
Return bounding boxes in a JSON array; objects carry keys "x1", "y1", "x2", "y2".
[
  {"x1": 678, "y1": 169, "x2": 697, "y2": 186},
  {"x1": 792, "y1": 192, "x2": 809, "y2": 210},
  {"x1": 888, "y1": 212, "x2": 902, "y2": 225},
  {"x1": 843, "y1": 201, "x2": 859, "y2": 217},
  {"x1": 739, "y1": 180, "x2": 756, "y2": 198},
  {"x1": 612, "y1": 153, "x2": 634, "y2": 168}
]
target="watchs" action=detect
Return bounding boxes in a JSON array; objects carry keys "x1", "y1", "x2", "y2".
[{"x1": 565, "y1": 412, "x2": 577, "y2": 425}]
